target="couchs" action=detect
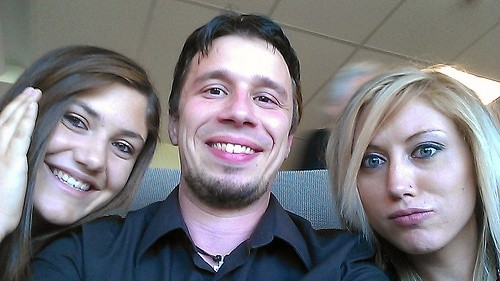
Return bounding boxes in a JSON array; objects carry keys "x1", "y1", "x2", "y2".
[{"x1": 107, "y1": 168, "x2": 341, "y2": 230}]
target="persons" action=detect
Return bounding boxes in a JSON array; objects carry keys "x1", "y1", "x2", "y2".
[
  {"x1": 0, "y1": 43, "x2": 162, "y2": 281},
  {"x1": 23, "y1": 12, "x2": 390, "y2": 281},
  {"x1": 323, "y1": 67, "x2": 500, "y2": 281}
]
